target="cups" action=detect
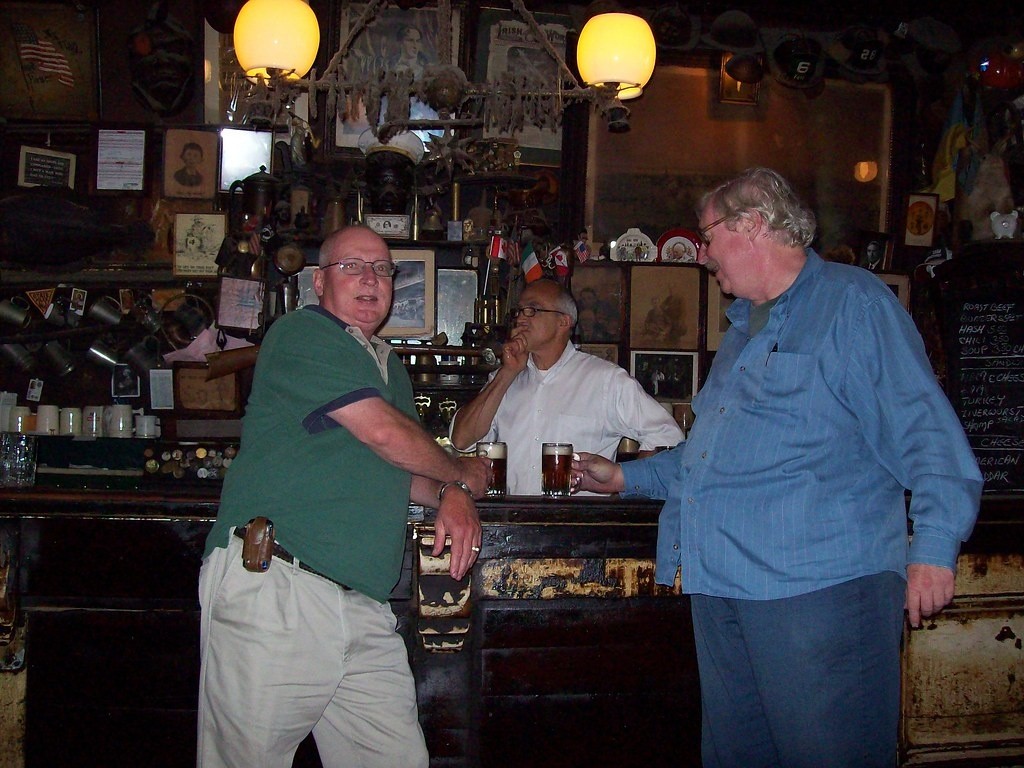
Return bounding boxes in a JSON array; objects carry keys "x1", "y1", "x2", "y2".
[
  {"x1": 540, "y1": 443, "x2": 582, "y2": 498},
  {"x1": 230, "y1": 166, "x2": 277, "y2": 229},
  {"x1": 476, "y1": 441, "x2": 508, "y2": 501},
  {"x1": 9, "y1": 404, "x2": 158, "y2": 439},
  {"x1": 0, "y1": 289, "x2": 206, "y2": 382},
  {"x1": 0, "y1": 433, "x2": 37, "y2": 488}
]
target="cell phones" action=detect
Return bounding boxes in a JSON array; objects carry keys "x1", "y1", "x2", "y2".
[{"x1": 243, "y1": 518, "x2": 273, "y2": 569}]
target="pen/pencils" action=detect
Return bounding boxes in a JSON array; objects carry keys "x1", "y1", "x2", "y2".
[{"x1": 764, "y1": 343, "x2": 779, "y2": 367}]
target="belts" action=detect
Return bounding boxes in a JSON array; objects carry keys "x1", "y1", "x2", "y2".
[{"x1": 235, "y1": 528, "x2": 354, "y2": 591}]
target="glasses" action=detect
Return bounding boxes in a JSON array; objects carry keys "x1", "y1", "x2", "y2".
[
  {"x1": 694, "y1": 215, "x2": 728, "y2": 247},
  {"x1": 510, "y1": 308, "x2": 566, "y2": 317},
  {"x1": 322, "y1": 258, "x2": 398, "y2": 277}
]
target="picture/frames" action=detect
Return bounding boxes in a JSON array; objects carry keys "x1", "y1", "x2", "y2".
[
  {"x1": 573, "y1": 262, "x2": 737, "y2": 406},
  {"x1": 172, "y1": 211, "x2": 229, "y2": 278},
  {"x1": 373, "y1": 248, "x2": 439, "y2": 342},
  {"x1": 172, "y1": 359, "x2": 245, "y2": 419},
  {"x1": 161, "y1": 127, "x2": 221, "y2": 200},
  {"x1": 719, "y1": 51, "x2": 761, "y2": 107},
  {"x1": 854, "y1": 191, "x2": 939, "y2": 317},
  {"x1": 217, "y1": 273, "x2": 268, "y2": 335},
  {"x1": 325, "y1": 0, "x2": 578, "y2": 170}
]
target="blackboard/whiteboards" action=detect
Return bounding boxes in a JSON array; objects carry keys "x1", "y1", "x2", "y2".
[{"x1": 949, "y1": 299, "x2": 1024, "y2": 499}]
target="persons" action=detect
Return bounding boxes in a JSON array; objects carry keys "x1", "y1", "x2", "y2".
[
  {"x1": 450, "y1": 278, "x2": 685, "y2": 498},
  {"x1": 862, "y1": 240, "x2": 883, "y2": 270},
  {"x1": 673, "y1": 243, "x2": 692, "y2": 261},
  {"x1": 196, "y1": 225, "x2": 493, "y2": 768},
  {"x1": 568, "y1": 167, "x2": 984, "y2": 768}
]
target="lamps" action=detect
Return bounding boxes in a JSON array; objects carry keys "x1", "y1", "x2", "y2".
[{"x1": 231, "y1": 0, "x2": 658, "y2": 179}]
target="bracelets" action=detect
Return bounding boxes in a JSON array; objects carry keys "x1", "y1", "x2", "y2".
[{"x1": 438, "y1": 481, "x2": 473, "y2": 500}]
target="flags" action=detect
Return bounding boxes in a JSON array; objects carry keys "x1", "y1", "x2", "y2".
[
  {"x1": 552, "y1": 244, "x2": 569, "y2": 276},
  {"x1": 490, "y1": 226, "x2": 520, "y2": 264},
  {"x1": 575, "y1": 241, "x2": 589, "y2": 263},
  {"x1": 521, "y1": 242, "x2": 543, "y2": 284}
]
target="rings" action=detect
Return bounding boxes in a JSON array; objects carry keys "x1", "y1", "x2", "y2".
[
  {"x1": 472, "y1": 546, "x2": 479, "y2": 552},
  {"x1": 490, "y1": 461, "x2": 494, "y2": 469}
]
